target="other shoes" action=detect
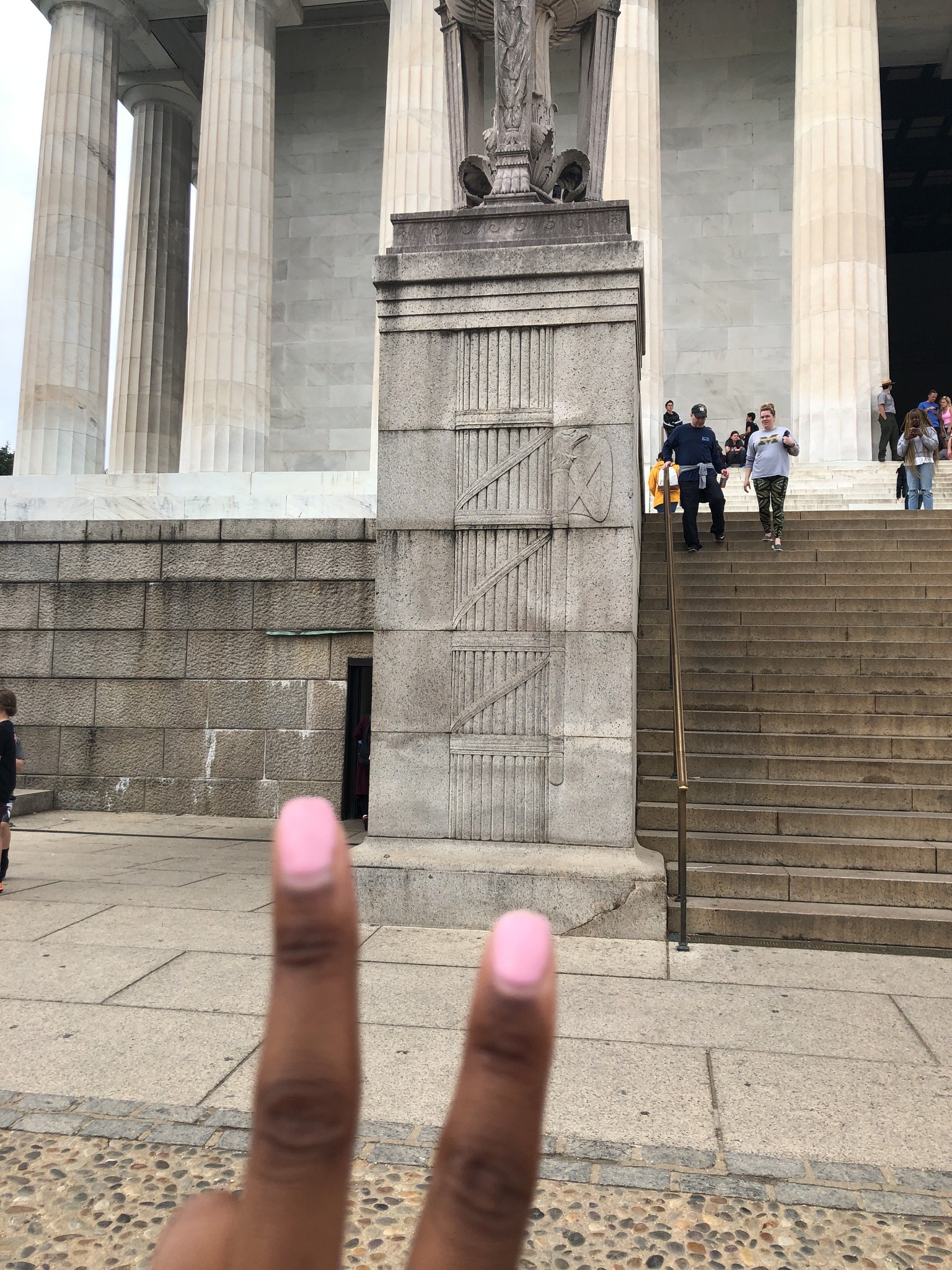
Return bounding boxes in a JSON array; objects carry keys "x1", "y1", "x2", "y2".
[
  {"x1": 895, "y1": 457, "x2": 904, "y2": 461},
  {"x1": 714, "y1": 534, "x2": 725, "y2": 542},
  {"x1": 761, "y1": 535, "x2": 773, "y2": 541},
  {"x1": 0, "y1": 882, "x2": 3, "y2": 892},
  {"x1": 739, "y1": 464, "x2": 745, "y2": 468},
  {"x1": 363, "y1": 815, "x2": 368, "y2": 830},
  {"x1": 726, "y1": 464, "x2": 732, "y2": 468},
  {"x1": 878, "y1": 458, "x2": 885, "y2": 462},
  {"x1": 687, "y1": 546, "x2": 699, "y2": 552},
  {"x1": 772, "y1": 541, "x2": 783, "y2": 551}
]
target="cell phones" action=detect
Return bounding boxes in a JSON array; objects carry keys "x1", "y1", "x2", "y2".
[
  {"x1": 934, "y1": 408, "x2": 937, "y2": 412},
  {"x1": 910, "y1": 427, "x2": 914, "y2": 431}
]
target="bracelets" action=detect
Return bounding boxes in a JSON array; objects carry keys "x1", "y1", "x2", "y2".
[
  {"x1": 743, "y1": 438, "x2": 745, "y2": 441},
  {"x1": 789, "y1": 443, "x2": 795, "y2": 448}
]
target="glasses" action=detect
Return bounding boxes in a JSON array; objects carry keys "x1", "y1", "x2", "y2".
[{"x1": 656, "y1": 458, "x2": 659, "y2": 462}]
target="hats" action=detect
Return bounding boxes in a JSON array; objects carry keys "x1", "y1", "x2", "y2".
[
  {"x1": 691, "y1": 403, "x2": 708, "y2": 418},
  {"x1": 879, "y1": 379, "x2": 896, "y2": 387}
]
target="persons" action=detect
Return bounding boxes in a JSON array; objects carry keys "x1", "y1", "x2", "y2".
[
  {"x1": 744, "y1": 402, "x2": 800, "y2": 551},
  {"x1": 725, "y1": 431, "x2": 746, "y2": 468},
  {"x1": 739, "y1": 412, "x2": 759, "y2": 451},
  {"x1": 648, "y1": 452, "x2": 679, "y2": 513},
  {"x1": 896, "y1": 408, "x2": 939, "y2": 510},
  {"x1": 663, "y1": 403, "x2": 729, "y2": 551},
  {"x1": 143, "y1": 795, "x2": 557, "y2": 1270},
  {"x1": 0, "y1": 684, "x2": 24, "y2": 894},
  {"x1": 663, "y1": 400, "x2": 683, "y2": 464},
  {"x1": 352, "y1": 714, "x2": 371, "y2": 830},
  {"x1": 918, "y1": 390, "x2": 952, "y2": 460},
  {"x1": 877, "y1": 378, "x2": 903, "y2": 461}
]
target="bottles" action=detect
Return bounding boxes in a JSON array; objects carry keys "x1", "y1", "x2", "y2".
[{"x1": 720, "y1": 470, "x2": 727, "y2": 488}]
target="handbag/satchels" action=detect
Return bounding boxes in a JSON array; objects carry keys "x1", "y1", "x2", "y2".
[{"x1": 783, "y1": 430, "x2": 792, "y2": 455}]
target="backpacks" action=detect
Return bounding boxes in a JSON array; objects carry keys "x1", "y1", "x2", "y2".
[
  {"x1": 359, "y1": 716, "x2": 371, "y2": 770},
  {"x1": 751, "y1": 423, "x2": 759, "y2": 434},
  {"x1": 657, "y1": 466, "x2": 679, "y2": 492}
]
